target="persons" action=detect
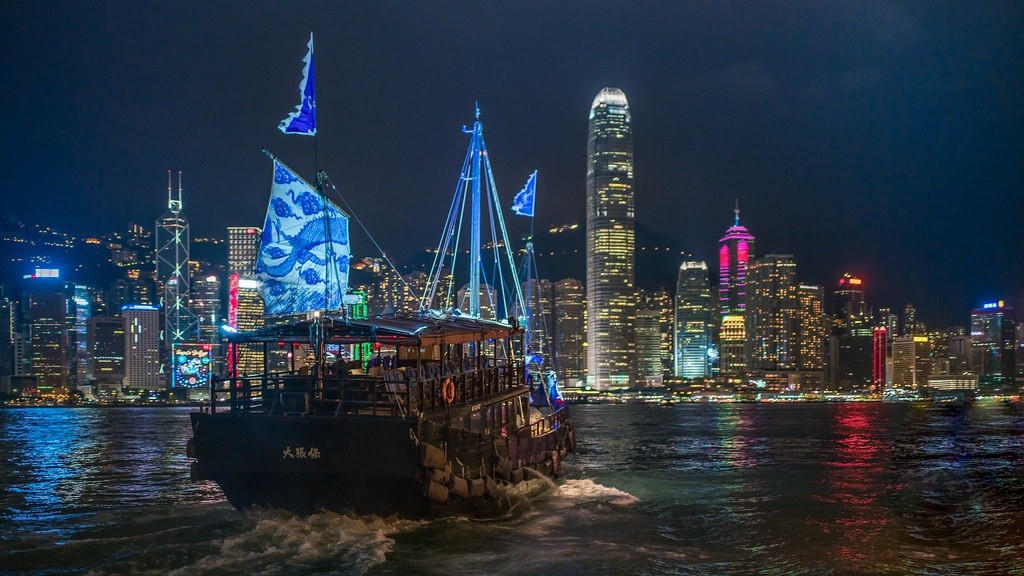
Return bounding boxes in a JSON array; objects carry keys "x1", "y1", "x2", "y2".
[{"x1": 313, "y1": 353, "x2": 347, "y2": 378}]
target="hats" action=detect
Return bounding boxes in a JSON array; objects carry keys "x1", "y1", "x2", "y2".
[{"x1": 335, "y1": 354, "x2": 342, "y2": 359}]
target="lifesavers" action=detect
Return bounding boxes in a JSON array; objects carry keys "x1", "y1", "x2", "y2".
[{"x1": 441, "y1": 377, "x2": 456, "y2": 405}]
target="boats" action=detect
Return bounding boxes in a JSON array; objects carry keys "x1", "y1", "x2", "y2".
[{"x1": 187, "y1": 94, "x2": 575, "y2": 525}]
